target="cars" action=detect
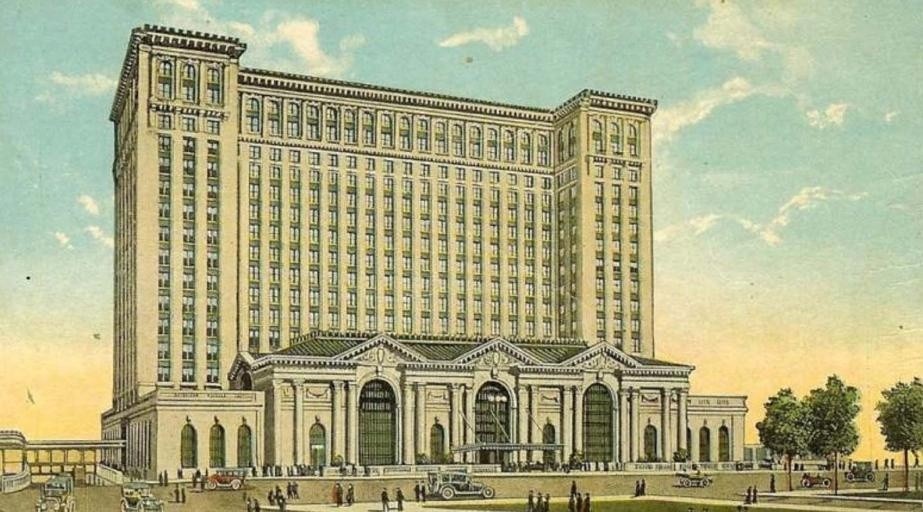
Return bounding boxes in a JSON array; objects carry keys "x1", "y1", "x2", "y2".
[
  {"x1": 801, "y1": 472, "x2": 832, "y2": 487},
  {"x1": 428, "y1": 469, "x2": 494, "y2": 500},
  {"x1": 845, "y1": 462, "x2": 876, "y2": 483},
  {"x1": 36, "y1": 475, "x2": 76, "y2": 512},
  {"x1": 120, "y1": 482, "x2": 165, "y2": 512},
  {"x1": 676, "y1": 465, "x2": 713, "y2": 488},
  {"x1": 208, "y1": 469, "x2": 248, "y2": 490}
]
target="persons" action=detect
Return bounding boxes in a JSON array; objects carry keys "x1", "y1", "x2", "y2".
[
  {"x1": 879, "y1": 471, "x2": 890, "y2": 490},
  {"x1": 745, "y1": 487, "x2": 751, "y2": 504},
  {"x1": 574, "y1": 493, "x2": 583, "y2": 512},
  {"x1": 570, "y1": 480, "x2": 577, "y2": 497},
  {"x1": 534, "y1": 491, "x2": 543, "y2": 512},
  {"x1": 640, "y1": 478, "x2": 645, "y2": 496},
  {"x1": 582, "y1": 493, "x2": 591, "y2": 512},
  {"x1": 567, "y1": 495, "x2": 576, "y2": 512},
  {"x1": 131, "y1": 458, "x2": 428, "y2": 512},
  {"x1": 500, "y1": 459, "x2": 626, "y2": 474},
  {"x1": 526, "y1": 490, "x2": 535, "y2": 512},
  {"x1": 543, "y1": 492, "x2": 550, "y2": 512},
  {"x1": 634, "y1": 480, "x2": 640, "y2": 497},
  {"x1": 751, "y1": 485, "x2": 757, "y2": 503},
  {"x1": 768, "y1": 472, "x2": 776, "y2": 494}
]
